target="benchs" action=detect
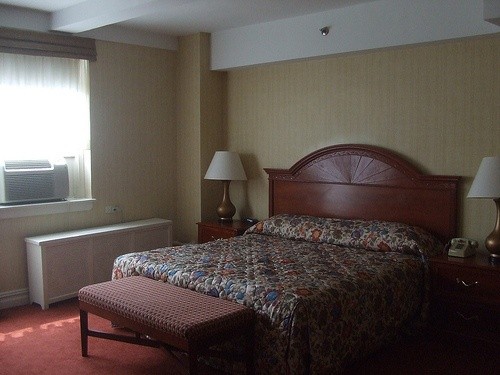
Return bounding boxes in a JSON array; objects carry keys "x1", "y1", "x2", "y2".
[{"x1": 79, "y1": 276, "x2": 255, "y2": 375}]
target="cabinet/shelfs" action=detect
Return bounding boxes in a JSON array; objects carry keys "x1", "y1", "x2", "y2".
[{"x1": 26, "y1": 217, "x2": 174, "y2": 309}]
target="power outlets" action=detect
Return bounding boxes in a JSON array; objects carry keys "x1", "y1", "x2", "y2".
[{"x1": 104, "y1": 206, "x2": 120, "y2": 214}]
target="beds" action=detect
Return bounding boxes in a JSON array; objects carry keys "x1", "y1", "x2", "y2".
[{"x1": 112, "y1": 144, "x2": 461, "y2": 375}]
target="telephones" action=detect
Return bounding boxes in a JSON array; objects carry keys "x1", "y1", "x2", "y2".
[{"x1": 448, "y1": 238, "x2": 479, "y2": 257}]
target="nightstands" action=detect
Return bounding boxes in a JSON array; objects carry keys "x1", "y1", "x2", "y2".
[
  {"x1": 428, "y1": 252, "x2": 500, "y2": 351},
  {"x1": 195, "y1": 220, "x2": 257, "y2": 244}
]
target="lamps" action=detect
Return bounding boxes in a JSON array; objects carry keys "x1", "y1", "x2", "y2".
[
  {"x1": 467, "y1": 157, "x2": 500, "y2": 256},
  {"x1": 204, "y1": 151, "x2": 249, "y2": 220}
]
